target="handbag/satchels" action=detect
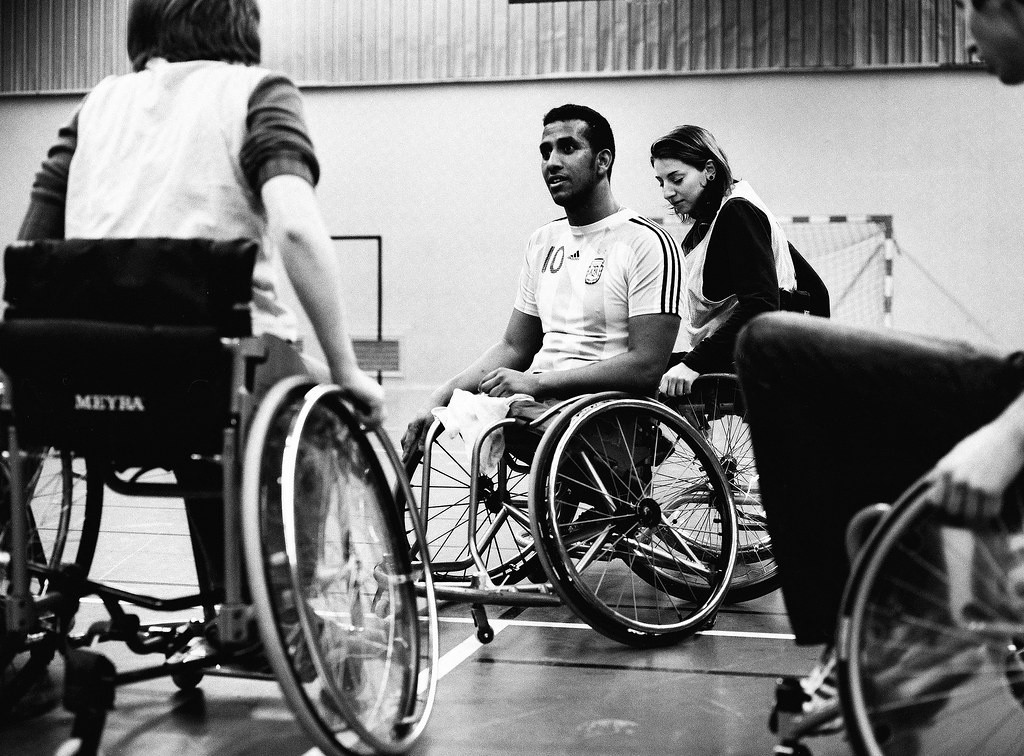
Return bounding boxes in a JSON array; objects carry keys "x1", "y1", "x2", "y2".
[{"x1": 778, "y1": 240, "x2": 830, "y2": 318}]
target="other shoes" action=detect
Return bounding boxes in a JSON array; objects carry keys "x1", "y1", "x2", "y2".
[
  {"x1": 765, "y1": 613, "x2": 985, "y2": 743},
  {"x1": 204, "y1": 602, "x2": 342, "y2": 675}
]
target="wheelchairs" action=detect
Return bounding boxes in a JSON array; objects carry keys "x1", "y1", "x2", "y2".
[
  {"x1": 1, "y1": 233, "x2": 441, "y2": 756},
  {"x1": 780, "y1": 465, "x2": 1024, "y2": 756},
  {"x1": 393, "y1": 365, "x2": 783, "y2": 649}
]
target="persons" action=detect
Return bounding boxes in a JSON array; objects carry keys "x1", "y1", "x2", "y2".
[
  {"x1": 735, "y1": 308, "x2": 1023, "y2": 736},
  {"x1": 649, "y1": 124, "x2": 802, "y2": 411},
  {"x1": 17, "y1": 0, "x2": 390, "y2": 667},
  {"x1": 397, "y1": 104, "x2": 684, "y2": 465}
]
